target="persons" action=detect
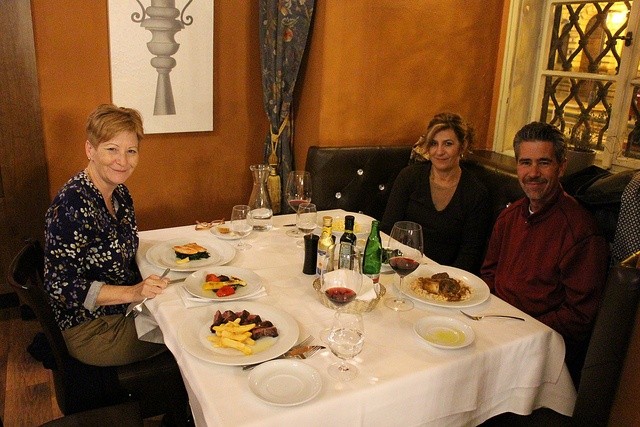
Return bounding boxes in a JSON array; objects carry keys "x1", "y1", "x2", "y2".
[
  {"x1": 45, "y1": 105, "x2": 169, "y2": 367},
  {"x1": 379, "y1": 112, "x2": 490, "y2": 274},
  {"x1": 481, "y1": 123, "x2": 608, "y2": 326}
]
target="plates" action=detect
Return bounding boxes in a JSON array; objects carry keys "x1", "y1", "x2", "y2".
[
  {"x1": 311, "y1": 210, "x2": 378, "y2": 236},
  {"x1": 378, "y1": 241, "x2": 422, "y2": 271},
  {"x1": 396, "y1": 264, "x2": 490, "y2": 308},
  {"x1": 413, "y1": 315, "x2": 474, "y2": 350},
  {"x1": 145, "y1": 235, "x2": 237, "y2": 271},
  {"x1": 211, "y1": 222, "x2": 252, "y2": 239},
  {"x1": 184, "y1": 265, "x2": 264, "y2": 301},
  {"x1": 179, "y1": 301, "x2": 299, "y2": 366},
  {"x1": 248, "y1": 358, "x2": 323, "y2": 407}
]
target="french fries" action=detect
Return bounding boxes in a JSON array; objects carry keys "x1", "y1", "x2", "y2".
[{"x1": 208, "y1": 316, "x2": 256, "y2": 355}]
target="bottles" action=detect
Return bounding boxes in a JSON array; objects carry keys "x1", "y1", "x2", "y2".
[
  {"x1": 338, "y1": 215, "x2": 357, "y2": 270},
  {"x1": 362, "y1": 221, "x2": 382, "y2": 284},
  {"x1": 315, "y1": 216, "x2": 335, "y2": 276},
  {"x1": 302, "y1": 231, "x2": 320, "y2": 274},
  {"x1": 246, "y1": 165, "x2": 274, "y2": 231}
]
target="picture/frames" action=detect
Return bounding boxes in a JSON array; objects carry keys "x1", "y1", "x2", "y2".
[{"x1": 107, "y1": 1, "x2": 215, "y2": 135}]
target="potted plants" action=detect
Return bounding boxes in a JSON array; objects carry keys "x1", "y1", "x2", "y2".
[{"x1": 566, "y1": 124, "x2": 596, "y2": 174}]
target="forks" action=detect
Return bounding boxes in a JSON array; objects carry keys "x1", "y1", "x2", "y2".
[
  {"x1": 242, "y1": 335, "x2": 314, "y2": 371},
  {"x1": 124, "y1": 268, "x2": 170, "y2": 319},
  {"x1": 295, "y1": 345, "x2": 320, "y2": 359},
  {"x1": 461, "y1": 311, "x2": 525, "y2": 321}
]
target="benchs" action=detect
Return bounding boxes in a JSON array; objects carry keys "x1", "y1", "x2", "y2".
[
  {"x1": 303, "y1": 145, "x2": 431, "y2": 283},
  {"x1": 460, "y1": 148, "x2": 527, "y2": 279}
]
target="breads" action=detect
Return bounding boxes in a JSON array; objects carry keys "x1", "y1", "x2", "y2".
[{"x1": 173, "y1": 242, "x2": 211, "y2": 260}]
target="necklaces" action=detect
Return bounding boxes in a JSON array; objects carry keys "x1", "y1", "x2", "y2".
[{"x1": 85, "y1": 168, "x2": 116, "y2": 214}]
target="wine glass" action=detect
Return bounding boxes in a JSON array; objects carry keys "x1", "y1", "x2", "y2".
[
  {"x1": 384, "y1": 221, "x2": 424, "y2": 311},
  {"x1": 327, "y1": 309, "x2": 365, "y2": 381},
  {"x1": 230, "y1": 205, "x2": 253, "y2": 249},
  {"x1": 286, "y1": 171, "x2": 313, "y2": 236},
  {"x1": 296, "y1": 203, "x2": 317, "y2": 247},
  {"x1": 321, "y1": 243, "x2": 363, "y2": 345}
]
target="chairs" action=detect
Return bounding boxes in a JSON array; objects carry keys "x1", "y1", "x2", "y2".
[{"x1": 7, "y1": 237, "x2": 171, "y2": 420}]
